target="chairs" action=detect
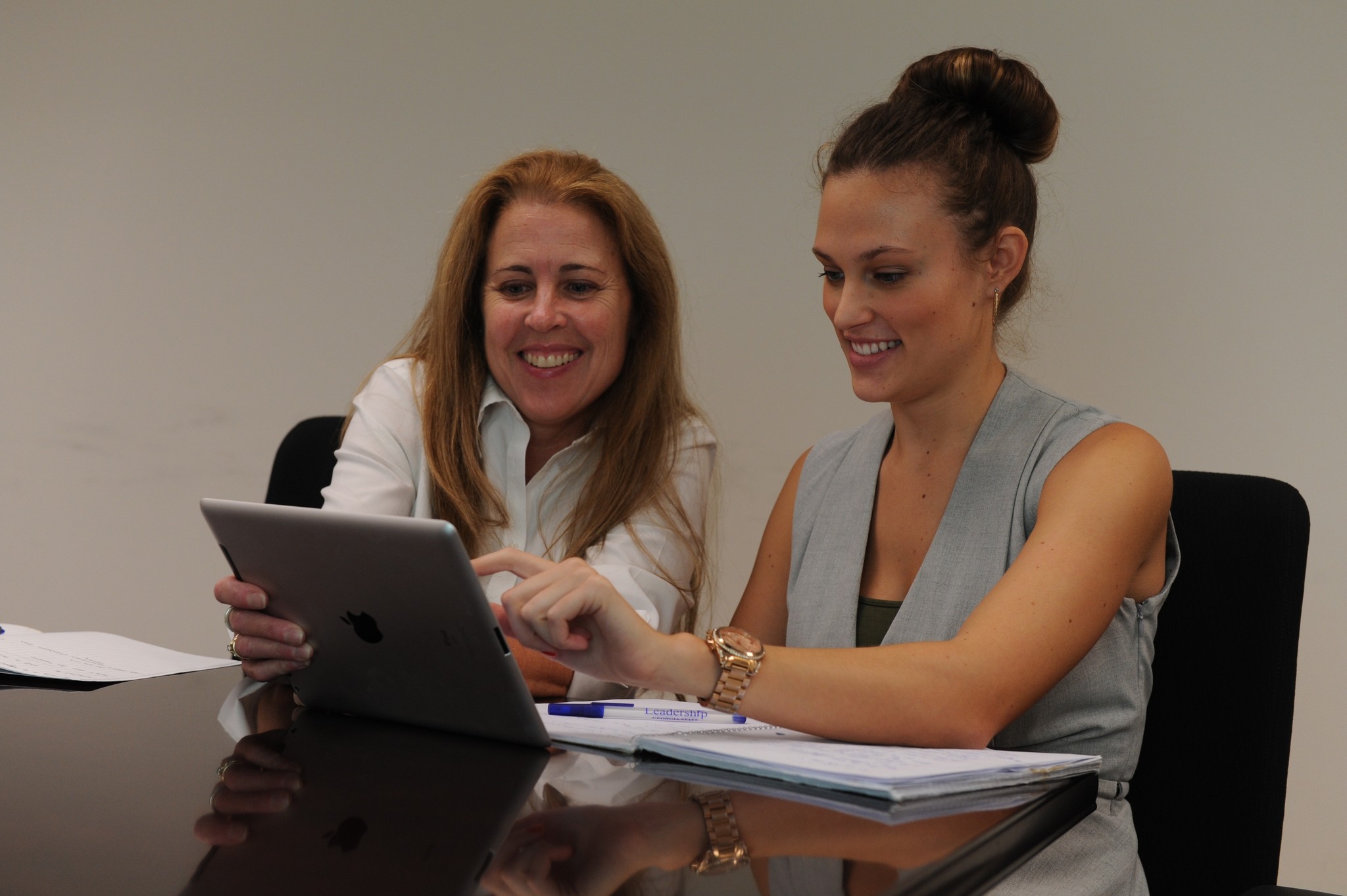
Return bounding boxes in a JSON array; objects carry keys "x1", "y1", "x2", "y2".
[{"x1": 1127, "y1": 471, "x2": 1338, "y2": 893}]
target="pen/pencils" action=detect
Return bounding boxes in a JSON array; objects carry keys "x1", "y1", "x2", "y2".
[{"x1": 546, "y1": 701, "x2": 748, "y2": 725}]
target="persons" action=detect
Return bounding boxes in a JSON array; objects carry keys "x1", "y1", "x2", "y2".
[
  {"x1": 464, "y1": 46, "x2": 1182, "y2": 895},
  {"x1": 476, "y1": 784, "x2": 1021, "y2": 896},
  {"x1": 191, "y1": 671, "x2": 719, "y2": 896},
  {"x1": 212, "y1": 151, "x2": 722, "y2": 702}
]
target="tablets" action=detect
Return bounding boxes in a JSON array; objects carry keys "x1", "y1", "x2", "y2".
[
  {"x1": 198, "y1": 496, "x2": 552, "y2": 752},
  {"x1": 188, "y1": 715, "x2": 551, "y2": 896}
]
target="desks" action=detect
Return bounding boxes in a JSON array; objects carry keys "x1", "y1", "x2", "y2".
[{"x1": 0, "y1": 634, "x2": 1099, "y2": 896}]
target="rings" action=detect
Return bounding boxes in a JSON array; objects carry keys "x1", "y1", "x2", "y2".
[
  {"x1": 227, "y1": 634, "x2": 253, "y2": 660},
  {"x1": 217, "y1": 757, "x2": 241, "y2": 784},
  {"x1": 224, "y1": 606, "x2": 239, "y2": 635},
  {"x1": 208, "y1": 781, "x2": 226, "y2": 816}
]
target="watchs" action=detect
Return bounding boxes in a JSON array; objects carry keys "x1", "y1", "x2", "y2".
[
  {"x1": 698, "y1": 625, "x2": 771, "y2": 716},
  {"x1": 688, "y1": 792, "x2": 751, "y2": 879}
]
